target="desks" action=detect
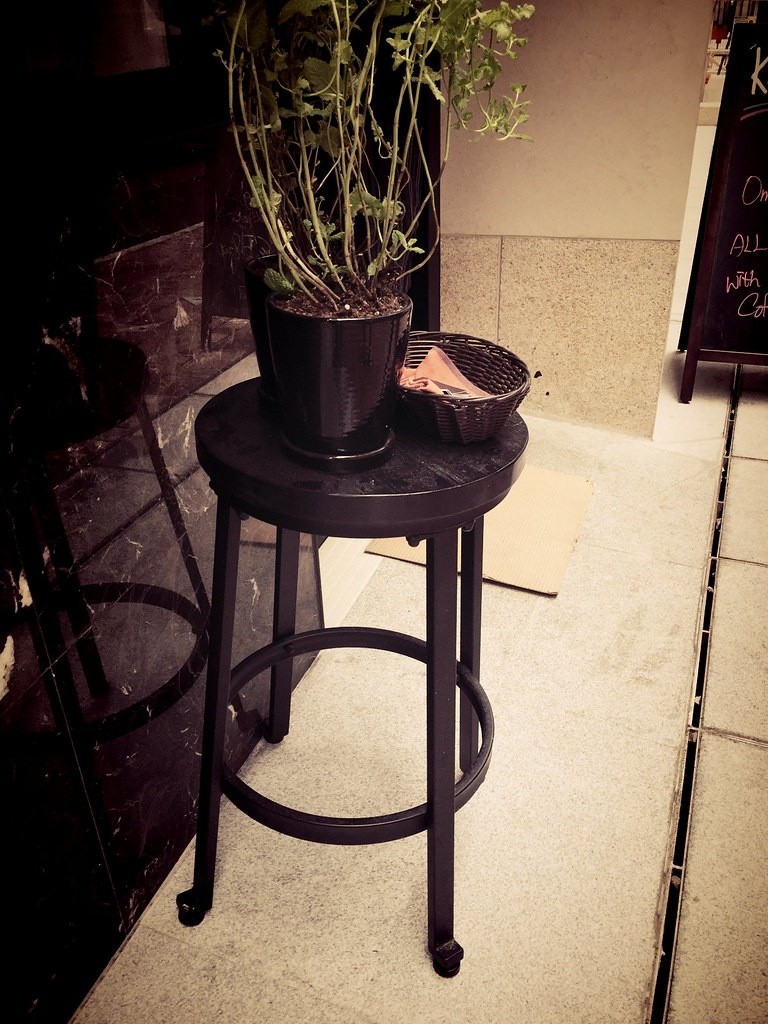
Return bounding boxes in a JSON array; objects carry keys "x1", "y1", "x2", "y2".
[{"x1": 176, "y1": 378, "x2": 528, "y2": 978}]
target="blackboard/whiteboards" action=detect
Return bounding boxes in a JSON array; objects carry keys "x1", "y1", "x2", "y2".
[{"x1": 674, "y1": 17, "x2": 768, "y2": 368}]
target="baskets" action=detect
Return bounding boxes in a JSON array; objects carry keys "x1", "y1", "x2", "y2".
[{"x1": 399, "y1": 331, "x2": 532, "y2": 446}]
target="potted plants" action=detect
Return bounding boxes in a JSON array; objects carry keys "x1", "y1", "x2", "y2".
[{"x1": 202, "y1": 0, "x2": 537, "y2": 476}]
